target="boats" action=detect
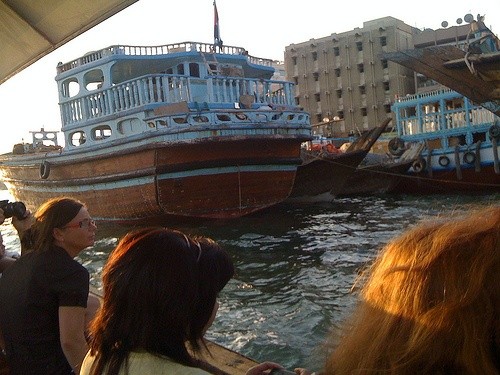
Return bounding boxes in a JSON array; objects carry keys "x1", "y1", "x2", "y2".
[
  {"x1": 389, "y1": 90, "x2": 499, "y2": 176},
  {"x1": 297, "y1": 111, "x2": 427, "y2": 200},
  {"x1": 379, "y1": 23, "x2": 500, "y2": 120},
  {"x1": 1, "y1": 42, "x2": 317, "y2": 226}
]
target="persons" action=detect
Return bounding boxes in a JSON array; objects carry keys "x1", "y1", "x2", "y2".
[
  {"x1": 76, "y1": 227, "x2": 317, "y2": 375},
  {"x1": 322, "y1": 202, "x2": 500, "y2": 374},
  {"x1": 0, "y1": 197, "x2": 97, "y2": 375}
]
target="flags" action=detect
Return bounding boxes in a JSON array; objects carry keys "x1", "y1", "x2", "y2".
[{"x1": 213, "y1": 5, "x2": 224, "y2": 47}]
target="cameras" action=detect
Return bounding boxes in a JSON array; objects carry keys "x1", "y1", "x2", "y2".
[{"x1": 0, "y1": 200, "x2": 27, "y2": 218}]
